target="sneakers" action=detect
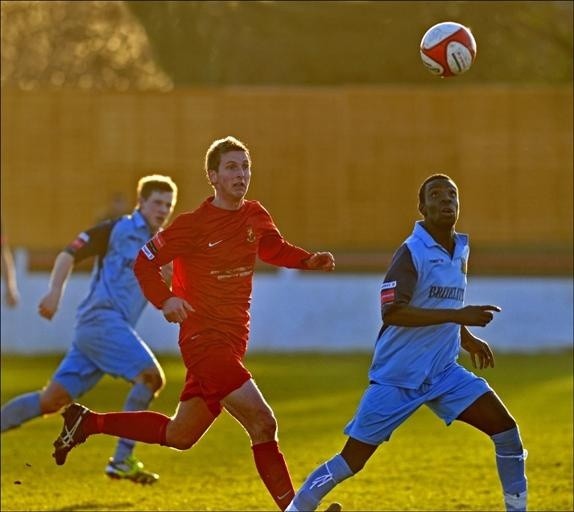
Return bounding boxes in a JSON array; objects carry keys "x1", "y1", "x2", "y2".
[
  {"x1": 105, "y1": 454, "x2": 160, "y2": 483},
  {"x1": 54, "y1": 403, "x2": 90, "y2": 465},
  {"x1": 323, "y1": 503, "x2": 341, "y2": 512}
]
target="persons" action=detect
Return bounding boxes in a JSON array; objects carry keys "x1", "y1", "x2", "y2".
[
  {"x1": 0, "y1": 210, "x2": 20, "y2": 305},
  {"x1": 284, "y1": 173, "x2": 527, "y2": 512},
  {"x1": 52, "y1": 136, "x2": 341, "y2": 512},
  {"x1": 0, "y1": 175, "x2": 178, "y2": 483}
]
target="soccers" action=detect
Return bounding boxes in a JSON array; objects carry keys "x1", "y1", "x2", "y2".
[{"x1": 421, "y1": 21, "x2": 478, "y2": 78}]
